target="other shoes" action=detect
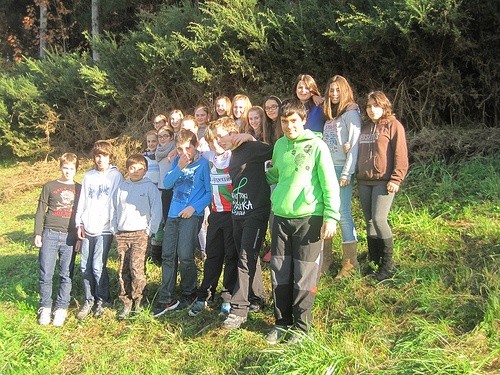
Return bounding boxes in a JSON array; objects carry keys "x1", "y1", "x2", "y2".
[
  {"x1": 266, "y1": 325, "x2": 290, "y2": 344},
  {"x1": 262, "y1": 247, "x2": 271, "y2": 263},
  {"x1": 288, "y1": 332, "x2": 305, "y2": 344}
]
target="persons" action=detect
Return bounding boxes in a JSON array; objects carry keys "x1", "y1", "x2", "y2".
[
  {"x1": 344, "y1": 92, "x2": 408, "y2": 281},
  {"x1": 318, "y1": 75, "x2": 361, "y2": 280},
  {"x1": 148, "y1": 128, "x2": 212, "y2": 317},
  {"x1": 110, "y1": 154, "x2": 162, "y2": 320},
  {"x1": 263, "y1": 98, "x2": 340, "y2": 346},
  {"x1": 152, "y1": 74, "x2": 358, "y2": 263},
  {"x1": 34, "y1": 152, "x2": 82, "y2": 328},
  {"x1": 167, "y1": 117, "x2": 274, "y2": 330},
  {"x1": 75, "y1": 141, "x2": 125, "y2": 320},
  {"x1": 142, "y1": 125, "x2": 178, "y2": 257}
]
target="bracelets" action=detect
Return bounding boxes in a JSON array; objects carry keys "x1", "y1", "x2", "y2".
[{"x1": 76, "y1": 238, "x2": 82, "y2": 241}]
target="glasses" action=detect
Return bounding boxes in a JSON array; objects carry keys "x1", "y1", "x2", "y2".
[
  {"x1": 264, "y1": 105, "x2": 280, "y2": 112},
  {"x1": 157, "y1": 134, "x2": 171, "y2": 139}
]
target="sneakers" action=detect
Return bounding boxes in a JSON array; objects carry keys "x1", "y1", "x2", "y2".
[
  {"x1": 248, "y1": 300, "x2": 269, "y2": 312},
  {"x1": 188, "y1": 300, "x2": 207, "y2": 317},
  {"x1": 94, "y1": 300, "x2": 108, "y2": 318},
  {"x1": 53, "y1": 308, "x2": 67, "y2": 326},
  {"x1": 77, "y1": 300, "x2": 95, "y2": 320},
  {"x1": 133, "y1": 300, "x2": 146, "y2": 316},
  {"x1": 118, "y1": 303, "x2": 132, "y2": 320},
  {"x1": 176, "y1": 293, "x2": 198, "y2": 312},
  {"x1": 37, "y1": 307, "x2": 51, "y2": 325},
  {"x1": 221, "y1": 313, "x2": 248, "y2": 330},
  {"x1": 149, "y1": 298, "x2": 180, "y2": 317},
  {"x1": 220, "y1": 302, "x2": 231, "y2": 315}
]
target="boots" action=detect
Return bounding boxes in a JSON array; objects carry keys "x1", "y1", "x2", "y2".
[
  {"x1": 372, "y1": 237, "x2": 395, "y2": 282},
  {"x1": 320, "y1": 238, "x2": 333, "y2": 278},
  {"x1": 363, "y1": 235, "x2": 380, "y2": 276},
  {"x1": 336, "y1": 241, "x2": 358, "y2": 279}
]
